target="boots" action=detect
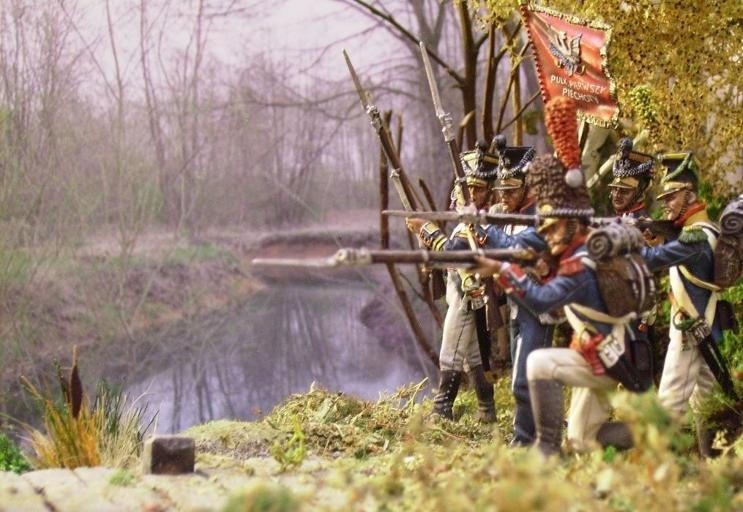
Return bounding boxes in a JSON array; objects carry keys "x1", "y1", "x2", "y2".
[
  {"x1": 526, "y1": 378, "x2": 564, "y2": 453},
  {"x1": 599, "y1": 422, "x2": 632, "y2": 449},
  {"x1": 695, "y1": 424, "x2": 722, "y2": 459},
  {"x1": 428, "y1": 371, "x2": 462, "y2": 422},
  {"x1": 466, "y1": 365, "x2": 497, "y2": 423},
  {"x1": 512, "y1": 379, "x2": 535, "y2": 448}
]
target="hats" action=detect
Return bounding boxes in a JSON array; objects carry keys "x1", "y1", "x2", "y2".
[
  {"x1": 607, "y1": 138, "x2": 655, "y2": 190},
  {"x1": 491, "y1": 135, "x2": 535, "y2": 191},
  {"x1": 521, "y1": 155, "x2": 595, "y2": 233},
  {"x1": 655, "y1": 152, "x2": 701, "y2": 200},
  {"x1": 453, "y1": 140, "x2": 498, "y2": 188}
]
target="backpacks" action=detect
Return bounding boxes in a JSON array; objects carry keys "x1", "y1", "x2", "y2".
[
  {"x1": 556, "y1": 224, "x2": 655, "y2": 321},
  {"x1": 676, "y1": 197, "x2": 742, "y2": 291}
]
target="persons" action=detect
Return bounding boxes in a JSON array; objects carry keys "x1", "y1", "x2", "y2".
[
  {"x1": 463, "y1": 147, "x2": 558, "y2": 450},
  {"x1": 609, "y1": 138, "x2": 659, "y2": 247},
  {"x1": 466, "y1": 151, "x2": 653, "y2": 460},
  {"x1": 403, "y1": 155, "x2": 499, "y2": 424},
  {"x1": 633, "y1": 151, "x2": 722, "y2": 427}
]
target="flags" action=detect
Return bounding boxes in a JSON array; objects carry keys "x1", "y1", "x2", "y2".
[{"x1": 528, "y1": 6, "x2": 620, "y2": 132}]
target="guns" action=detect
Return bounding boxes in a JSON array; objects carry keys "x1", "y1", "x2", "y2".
[
  {"x1": 383, "y1": 209, "x2": 679, "y2": 244},
  {"x1": 342, "y1": 49, "x2": 446, "y2": 298},
  {"x1": 419, "y1": 42, "x2": 503, "y2": 330},
  {"x1": 253, "y1": 249, "x2": 558, "y2": 280}
]
